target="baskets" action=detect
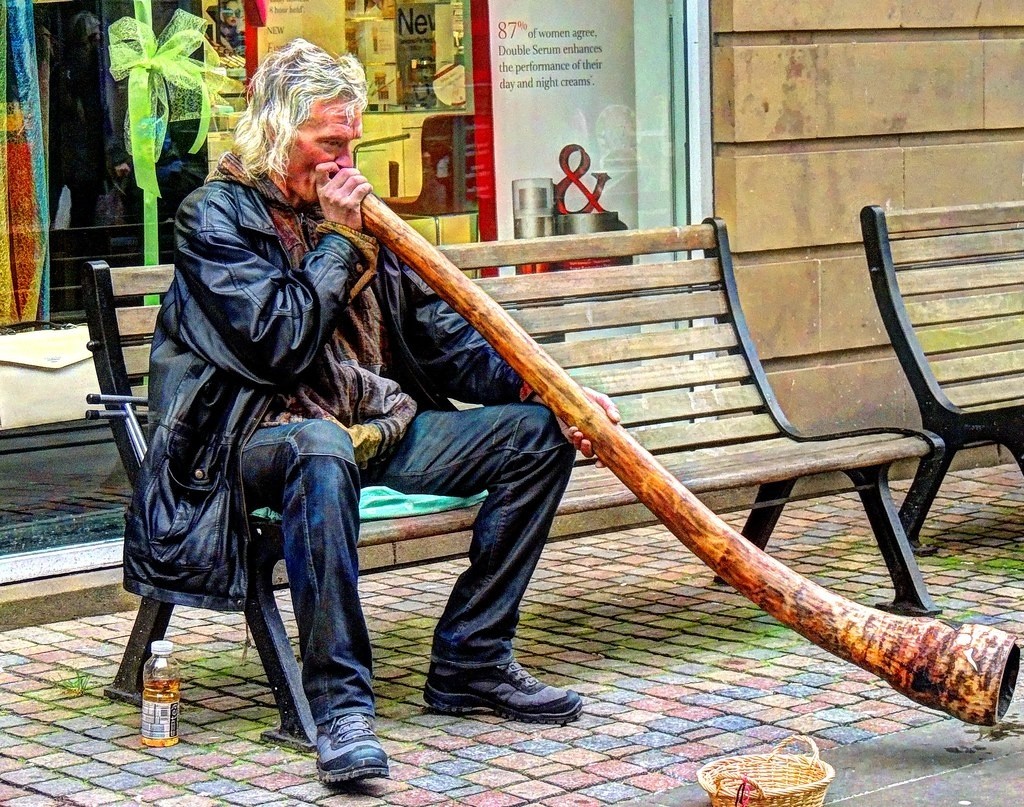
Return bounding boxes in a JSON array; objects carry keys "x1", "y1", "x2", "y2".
[{"x1": 696, "y1": 735, "x2": 835, "y2": 807}]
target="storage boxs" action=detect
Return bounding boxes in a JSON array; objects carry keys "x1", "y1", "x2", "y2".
[
  {"x1": 208, "y1": 112, "x2": 241, "y2": 132},
  {"x1": 355, "y1": 0, "x2": 401, "y2": 106}
]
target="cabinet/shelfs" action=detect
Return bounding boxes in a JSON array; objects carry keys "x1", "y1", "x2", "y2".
[{"x1": 206, "y1": 112, "x2": 476, "y2": 277}]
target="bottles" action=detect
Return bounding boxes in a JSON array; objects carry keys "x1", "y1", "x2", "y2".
[{"x1": 142, "y1": 641, "x2": 181, "y2": 746}]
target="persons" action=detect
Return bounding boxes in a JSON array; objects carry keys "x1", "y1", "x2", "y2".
[
  {"x1": 32, "y1": 0, "x2": 244, "y2": 306},
  {"x1": 120, "y1": 40, "x2": 619, "y2": 784}
]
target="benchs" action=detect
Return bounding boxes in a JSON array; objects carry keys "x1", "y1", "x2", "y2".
[
  {"x1": 860, "y1": 205, "x2": 1024, "y2": 557},
  {"x1": 82, "y1": 217, "x2": 945, "y2": 751},
  {"x1": 50, "y1": 221, "x2": 176, "y2": 320}
]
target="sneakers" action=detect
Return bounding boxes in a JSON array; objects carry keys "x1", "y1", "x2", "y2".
[
  {"x1": 316, "y1": 713, "x2": 389, "y2": 783},
  {"x1": 423, "y1": 658, "x2": 582, "y2": 724}
]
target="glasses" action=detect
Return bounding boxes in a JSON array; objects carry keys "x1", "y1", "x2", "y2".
[{"x1": 225, "y1": 8, "x2": 241, "y2": 19}]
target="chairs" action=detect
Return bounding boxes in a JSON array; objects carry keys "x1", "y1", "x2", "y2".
[{"x1": 381, "y1": 113, "x2": 478, "y2": 216}]
